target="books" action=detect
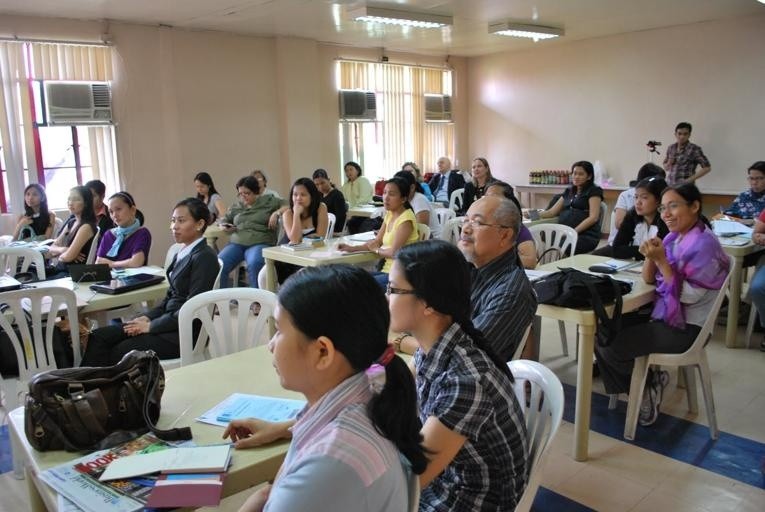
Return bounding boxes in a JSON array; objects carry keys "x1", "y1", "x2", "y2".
[
  {"x1": 144, "y1": 472, "x2": 224, "y2": 510},
  {"x1": 99, "y1": 445, "x2": 232, "y2": 482},
  {"x1": 72, "y1": 430, "x2": 179, "y2": 506}
]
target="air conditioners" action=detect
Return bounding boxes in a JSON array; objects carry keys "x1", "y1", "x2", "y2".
[
  {"x1": 43, "y1": 80, "x2": 112, "y2": 126},
  {"x1": 424, "y1": 93, "x2": 450, "y2": 120},
  {"x1": 339, "y1": 89, "x2": 376, "y2": 119}
]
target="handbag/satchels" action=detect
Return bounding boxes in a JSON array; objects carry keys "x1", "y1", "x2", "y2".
[
  {"x1": 526, "y1": 266, "x2": 633, "y2": 337},
  {"x1": 19, "y1": 347, "x2": 195, "y2": 453}
]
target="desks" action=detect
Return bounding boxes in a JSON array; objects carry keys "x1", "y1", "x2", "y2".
[
  {"x1": 202, "y1": 203, "x2": 385, "y2": 291},
  {"x1": 535, "y1": 254, "x2": 656, "y2": 462},
  {"x1": 2, "y1": 266, "x2": 170, "y2": 326},
  {"x1": 7, "y1": 345, "x2": 415, "y2": 512},
  {"x1": 719, "y1": 233, "x2": 765, "y2": 348},
  {"x1": 515, "y1": 185, "x2": 745, "y2": 210}
]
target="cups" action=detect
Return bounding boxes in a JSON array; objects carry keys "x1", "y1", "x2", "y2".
[{"x1": 528, "y1": 210, "x2": 538, "y2": 221}]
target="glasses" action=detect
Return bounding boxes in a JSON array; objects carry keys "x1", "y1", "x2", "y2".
[
  {"x1": 460, "y1": 213, "x2": 514, "y2": 230},
  {"x1": 657, "y1": 203, "x2": 692, "y2": 212},
  {"x1": 237, "y1": 190, "x2": 252, "y2": 197},
  {"x1": 384, "y1": 280, "x2": 424, "y2": 298}
]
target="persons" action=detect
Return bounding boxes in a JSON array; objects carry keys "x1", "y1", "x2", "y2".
[
  {"x1": 221, "y1": 261, "x2": 444, "y2": 511},
  {"x1": 747, "y1": 208, "x2": 764, "y2": 351},
  {"x1": 716, "y1": 160, "x2": 765, "y2": 327},
  {"x1": 1, "y1": 158, "x2": 732, "y2": 428},
  {"x1": 663, "y1": 120, "x2": 712, "y2": 187},
  {"x1": 382, "y1": 240, "x2": 533, "y2": 512}
]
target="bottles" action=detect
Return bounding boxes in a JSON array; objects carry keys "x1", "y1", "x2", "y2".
[{"x1": 528, "y1": 168, "x2": 573, "y2": 184}]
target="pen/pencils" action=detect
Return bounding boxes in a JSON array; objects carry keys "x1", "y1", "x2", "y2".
[
  {"x1": 217, "y1": 416, "x2": 231, "y2": 423},
  {"x1": 720, "y1": 205, "x2": 724, "y2": 215},
  {"x1": 22, "y1": 286, "x2": 36, "y2": 288}
]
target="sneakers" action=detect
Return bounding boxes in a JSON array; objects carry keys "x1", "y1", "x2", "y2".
[{"x1": 637, "y1": 370, "x2": 669, "y2": 427}]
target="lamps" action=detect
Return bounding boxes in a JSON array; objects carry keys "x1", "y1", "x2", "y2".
[
  {"x1": 489, "y1": 23, "x2": 560, "y2": 39},
  {"x1": 344, "y1": 7, "x2": 453, "y2": 28}
]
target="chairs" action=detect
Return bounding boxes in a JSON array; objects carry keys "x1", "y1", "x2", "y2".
[
  {"x1": 435, "y1": 188, "x2": 466, "y2": 244},
  {"x1": 528, "y1": 202, "x2": 607, "y2": 265},
  {"x1": 178, "y1": 287, "x2": 278, "y2": 368},
  {"x1": 0, "y1": 217, "x2": 81, "y2": 379},
  {"x1": 506, "y1": 359, "x2": 565, "y2": 512},
  {"x1": 622, "y1": 255, "x2": 736, "y2": 441}
]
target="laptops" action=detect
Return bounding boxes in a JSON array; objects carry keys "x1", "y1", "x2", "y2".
[
  {"x1": 89, "y1": 272, "x2": 165, "y2": 295},
  {"x1": 66, "y1": 263, "x2": 118, "y2": 281}
]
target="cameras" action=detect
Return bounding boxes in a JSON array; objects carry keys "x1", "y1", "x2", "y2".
[{"x1": 647, "y1": 141, "x2": 662, "y2": 147}]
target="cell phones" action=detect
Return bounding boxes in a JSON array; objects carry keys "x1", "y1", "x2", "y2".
[{"x1": 222, "y1": 223, "x2": 232, "y2": 228}]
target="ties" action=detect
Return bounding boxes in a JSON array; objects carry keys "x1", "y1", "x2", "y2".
[{"x1": 433, "y1": 176, "x2": 445, "y2": 197}]
